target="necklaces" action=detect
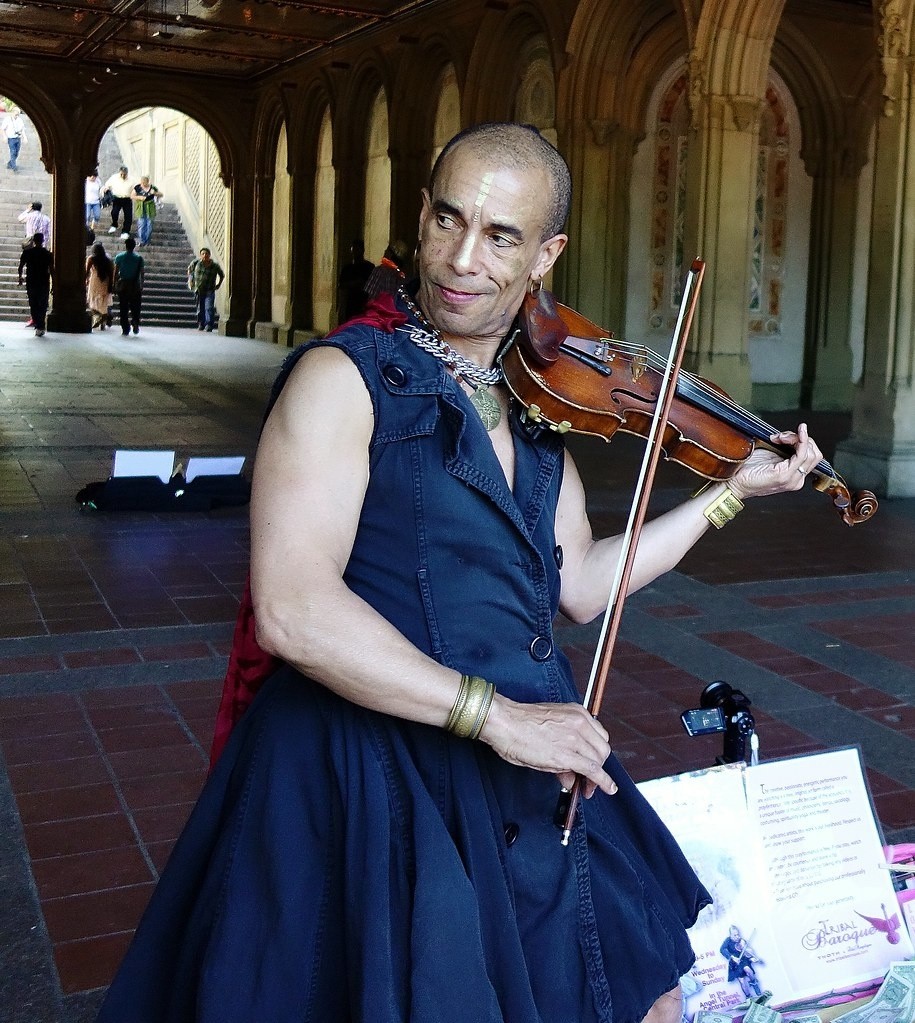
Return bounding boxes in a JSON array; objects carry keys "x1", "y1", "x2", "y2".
[{"x1": 394, "y1": 273, "x2": 507, "y2": 435}]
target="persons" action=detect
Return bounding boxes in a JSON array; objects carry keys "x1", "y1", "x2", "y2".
[
  {"x1": 187, "y1": 248, "x2": 225, "y2": 333},
  {"x1": 85, "y1": 174, "x2": 104, "y2": 231},
  {"x1": 85, "y1": 240, "x2": 115, "y2": 331},
  {"x1": 130, "y1": 174, "x2": 164, "y2": 249},
  {"x1": 720, "y1": 922, "x2": 771, "y2": 1008},
  {"x1": 104, "y1": 167, "x2": 136, "y2": 239},
  {"x1": 18, "y1": 233, "x2": 56, "y2": 338},
  {"x1": 113, "y1": 238, "x2": 145, "y2": 335},
  {"x1": 96, "y1": 122, "x2": 824, "y2": 1023},
  {"x1": 2, "y1": 107, "x2": 26, "y2": 173},
  {"x1": 17, "y1": 202, "x2": 52, "y2": 249}
]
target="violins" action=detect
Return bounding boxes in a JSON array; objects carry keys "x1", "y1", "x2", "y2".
[{"x1": 494, "y1": 285, "x2": 878, "y2": 527}]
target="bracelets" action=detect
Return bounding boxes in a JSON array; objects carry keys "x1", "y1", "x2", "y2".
[
  {"x1": 695, "y1": 478, "x2": 745, "y2": 532},
  {"x1": 447, "y1": 673, "x2": 498, "y2": 744}
]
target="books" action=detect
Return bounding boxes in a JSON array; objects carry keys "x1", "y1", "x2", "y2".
[{"x1": 635, "y1": 745, "x2": 911, "y2": 1017}]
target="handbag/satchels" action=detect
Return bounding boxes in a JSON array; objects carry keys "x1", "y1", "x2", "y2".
[{"x1": 114, "y1": 281, "x2": 126, "y2": 293}]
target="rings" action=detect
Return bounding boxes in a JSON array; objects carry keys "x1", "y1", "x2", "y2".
[{"x1": 800, "y1": 466, "x2": 808, "y2": 479}]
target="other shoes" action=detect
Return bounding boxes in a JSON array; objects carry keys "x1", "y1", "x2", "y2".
[
  {"x1": 198, "y1": 325, "x2": 204, "y2": 330},
  {"x1": 133, "y1": 324, "x2": 139, "y2": 334},
  {"x1": 36, "y1": 329, "x2": 44, "y2": 337},
  {"x1": 122, "y1": 329, "x2": 129, "y2": 335},
  {"x1": 108, "y1": 227, "x2": 116, "y2": 233},
  {"x1": 206, "y1": 326, "x2": 212, "y2": 332},
  {"x1": 92, "y1": 322, "x2": 99, "y2": 328},
  {"x1": 121, "y1": 233, "x2": 130, "y2": 239},
  {"x1": 107, "y1": 315, "x2": 113, "y2": 326}
]
